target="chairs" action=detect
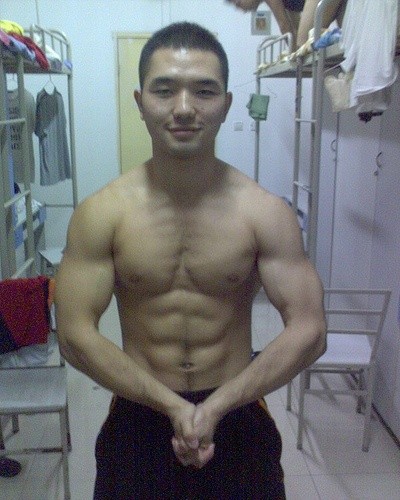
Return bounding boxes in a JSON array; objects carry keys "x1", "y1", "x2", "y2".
[
  {"x1": 286, "y1": 289, "x2": 392, "y2": 452},
  {"x1": 0, "y1": 278, "x2": 71, "y2": 500}
]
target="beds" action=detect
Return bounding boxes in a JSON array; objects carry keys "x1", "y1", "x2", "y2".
[
  {"x1": 1, "y1": 24, "x2": 79, "y2": 279},
  {"x1": 253, "y1": 1, "x2": 399, "y2": 269}
]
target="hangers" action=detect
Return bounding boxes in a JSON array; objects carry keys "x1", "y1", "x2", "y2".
[
  {"x1": 34, "y1": 74, "x2": 65, "y2": 105},
  {"x1": 234, "y1": 72, "x2": 279, "y2": 102},
  {"x1": 7, "y1": 74, "x2": 34, "y2": 104}
]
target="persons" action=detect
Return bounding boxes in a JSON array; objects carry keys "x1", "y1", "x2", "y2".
[
  {"x1": 225, "y1": 0, "x2": 342, "y2": 50},
  {"x1": 51, "y1": 22, "x2": 328, "y2": 500}
]
